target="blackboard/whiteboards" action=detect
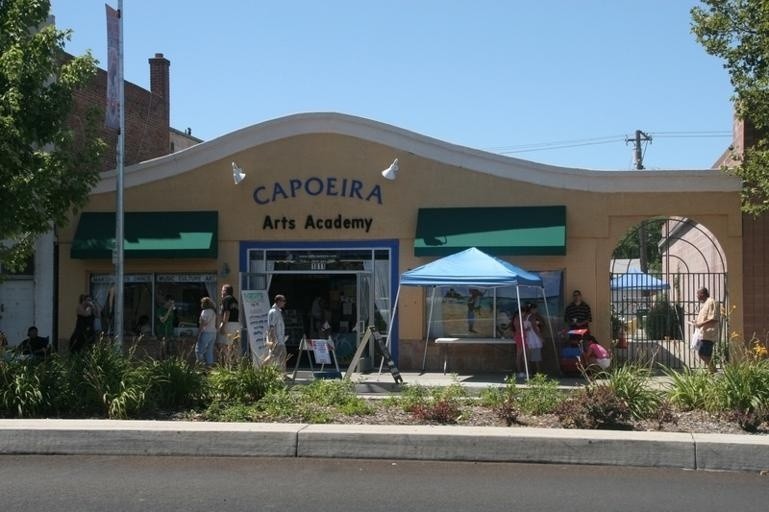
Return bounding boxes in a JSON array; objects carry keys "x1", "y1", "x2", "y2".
[
  {"x1": 241, "y1": 290, "x2": 271, "y2": 366},
  {"x1": 370, "y1": 326, "x2": 403, "y2": 384}
]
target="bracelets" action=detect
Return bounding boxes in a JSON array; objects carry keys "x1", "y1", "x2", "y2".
[{"x1": 220, "y1": 320, "x2": 224, "y2": 325}]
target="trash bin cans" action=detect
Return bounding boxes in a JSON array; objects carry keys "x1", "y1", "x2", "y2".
[{"x1": 637, "y1": 309, "x2": 649, "y2": 328}]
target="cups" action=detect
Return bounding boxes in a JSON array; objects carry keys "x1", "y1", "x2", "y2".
[{"x1": 573, "y1": 318, "x2": 578, "y2": 323}]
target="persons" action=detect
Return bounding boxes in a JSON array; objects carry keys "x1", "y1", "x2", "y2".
[
  {"x1": 305, "y1": 296, "x2": 333, "y2": 367},
  {"x1": 154, "y1": 294, "x2": 177, "y2": 361},
  {"x1": 68, "y1": 295, "x2": 101, "y2": 354},
  {"x1": 212, "y1": 284, "x2": 240, "y2": 368},
  {"x1": 194, "y1": 297, "x2": 217, "y2": 369},
  {"x1": 311, "y1": 291, "x2": 325, "y2": 324},
  {"x1": 15, "y1": 326, "x2": 48, "y2": 356},
  {"x1": 690, "y1": 287, "x2": 721, "y2": 374},
  {"x1": 466, "y1": 288, "x2": 612, "y2": 380},
  {"x1": 133, "y1": 314, "x2": 152, "y2": 336},
  {"x1": 260, "y1": 293, "x2": 289, "y2": 376}
]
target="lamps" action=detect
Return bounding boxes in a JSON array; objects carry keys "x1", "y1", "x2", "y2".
[
  {"x1": 381, "y1": 157, "x2": 400, "y2": 180},
  {"x1": 232, "y1": 161, "x2": 246, "y2": 184}
]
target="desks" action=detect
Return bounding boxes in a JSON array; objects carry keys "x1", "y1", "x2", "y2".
[{"x1": 434, "y1": 336, "x2": 517, "y2": 376}]
